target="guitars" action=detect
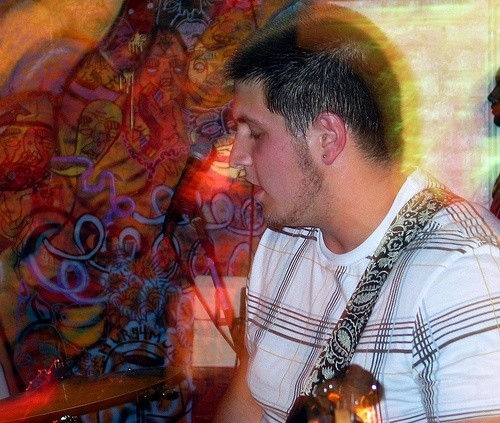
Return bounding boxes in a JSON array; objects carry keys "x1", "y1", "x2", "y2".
[{"x1": 286, "y1": 362, "x2": 384, "y2": 423}]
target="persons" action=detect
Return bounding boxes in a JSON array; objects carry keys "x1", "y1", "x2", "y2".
[{"x1": 211, "y1": 1, "x2": 498, "y2": 422}]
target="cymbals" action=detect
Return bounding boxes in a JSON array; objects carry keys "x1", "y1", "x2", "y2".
[{"x1": 0, "y1": 366, "x2": 180, "y2": 423}]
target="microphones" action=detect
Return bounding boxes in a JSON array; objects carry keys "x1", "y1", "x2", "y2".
[{"x1": 165, "y1": 136, "x2": 214, "y2": 236}]
